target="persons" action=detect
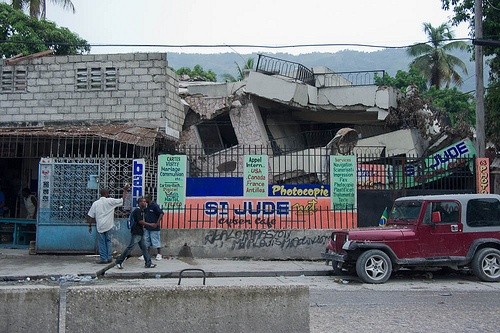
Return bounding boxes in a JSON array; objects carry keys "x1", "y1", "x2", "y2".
[
  {"x1": 135, "y1": 192, "x2": 165, "y2": 262},
  {"x1": 114, "y1": 197, "x2": 159, "y2": 270},
  {"x1": 86, "y1": 187, "x2": 127, "y2": 265},
  {"x1": 20, "y1": 187, "x2": 38, "y2": 246}
]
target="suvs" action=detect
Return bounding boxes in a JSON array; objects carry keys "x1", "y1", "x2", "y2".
[{"x1": 322, "y1": 194, "x2": 499, "y2": 282}]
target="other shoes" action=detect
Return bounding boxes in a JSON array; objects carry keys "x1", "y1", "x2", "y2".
[{"x1": 96, "y1": 259, "x2": 112, "y2": 264}]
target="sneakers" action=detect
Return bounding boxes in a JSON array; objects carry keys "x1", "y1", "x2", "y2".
[
  {"x1": 145, "y1": 264, "x2": 156, "y2": 268},
  {"x1": 114, "y1": 261, "x2": 123, "y2": 269},
  {"x1": 138, "y1": 255, "x2": 144, "y2": 261},
  {"x1": 155, "y1": 254, "x2": 162, "y2": 260}
]
